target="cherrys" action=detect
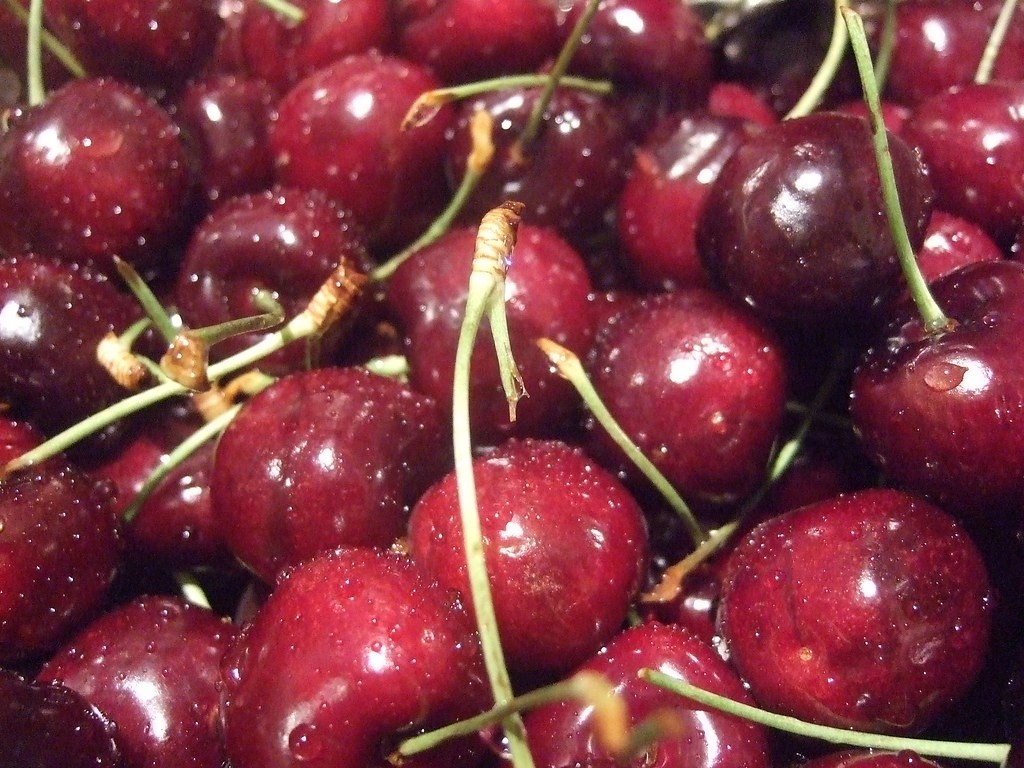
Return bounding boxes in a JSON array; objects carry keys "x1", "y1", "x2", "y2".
[{"x1": 1, "y1": 1, "x2": 1023, "y2": 768}]
